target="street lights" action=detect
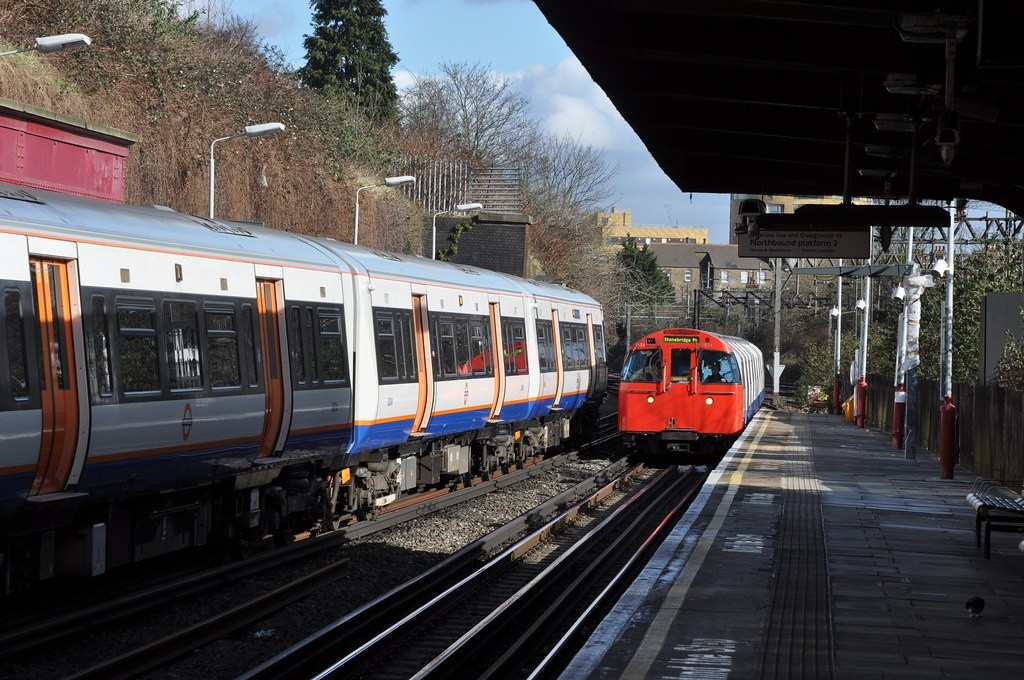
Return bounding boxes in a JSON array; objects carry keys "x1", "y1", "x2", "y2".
[
  {"x1": 432, "y1": 203, "x2": 483, "y2": 259},
  {"x1": 208, "y1": 122, "x2": 285, "y2": 221},
  {"x1": 353, "y1": 176, "x2": 415, "y2": 246}
]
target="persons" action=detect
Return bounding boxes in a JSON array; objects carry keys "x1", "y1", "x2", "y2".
[{"x1": 704, "y1": 361, "x2": 728, "y2": 383}]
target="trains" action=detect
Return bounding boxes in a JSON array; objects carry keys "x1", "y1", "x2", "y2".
[
  {"x1": 0, "y1": 96, "x2": 608, "y2": 658},
  {"x1": 616, "y1": 328, "x2": 765, "y2": 466}
]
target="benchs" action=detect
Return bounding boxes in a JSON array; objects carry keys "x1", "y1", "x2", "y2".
[{"x1": 965, "y1": 476, "x2": 1024, "y2": 559}]
